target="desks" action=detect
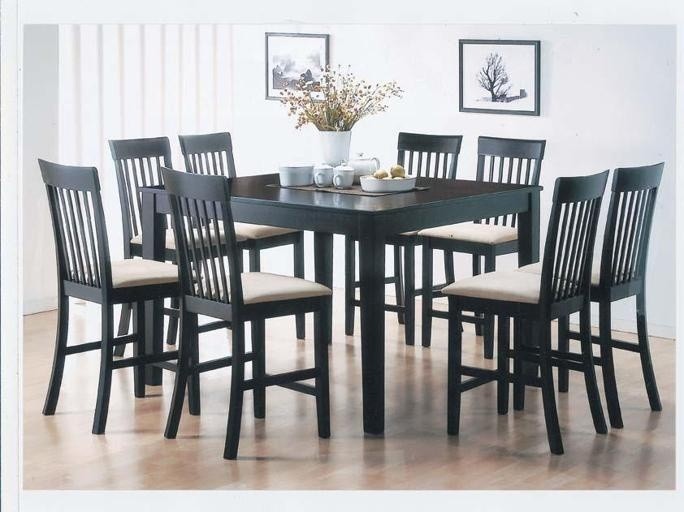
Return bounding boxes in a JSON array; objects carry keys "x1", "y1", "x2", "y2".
[{"x1": 131, "y1": 169, "x2": 549, "y2": 434}]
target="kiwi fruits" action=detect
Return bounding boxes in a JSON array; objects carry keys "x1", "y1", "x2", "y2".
[
  {"x1": 390, "y1": 165, "x2": 405, "y2": 177},
  {"x1": 373, "y1": 169, "x2": 388, "y2": 179}
]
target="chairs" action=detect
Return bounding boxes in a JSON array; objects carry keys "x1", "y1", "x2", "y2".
[
  {"x1": 145, "y1": 165, "x2": 336, "y2": 459},
  {"x1": 499, "y1": 161, "x2": 664, "y2": 430},
  {"x1": 340, "y1": 133, "x2": 466, "y2": 349},
  {"x1": 106, "y1": 133, "x2": 246, "y2": 364},
  {"x1": 400, "y1": 133, "x2": 549, "y2": 360},
  {"x1": 434, "y1": 166, "x2": 610, "y2": 457},
  {"x1": 38, "y1": 157, "x2": 202, "y2": 435},
  {"x1": 169, "y1": 131, "x2": 312, "y2": 341}
]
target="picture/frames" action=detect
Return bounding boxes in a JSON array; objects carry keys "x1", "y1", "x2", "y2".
[
  {"x1": 264, "y1": 32, "x2": 331, "y2": 105},
  {"x1": 457, "y1": 37, "x2": 541, "y2": 116}
]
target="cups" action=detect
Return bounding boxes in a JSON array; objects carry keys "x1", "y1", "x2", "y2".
[{"x1": 313, "y1": 160, "x2": 355, "y2": 189}]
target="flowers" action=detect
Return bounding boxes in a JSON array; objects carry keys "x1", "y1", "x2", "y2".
[{"x1": 280, "y1": 65, "x2": 403, "y2": 133}]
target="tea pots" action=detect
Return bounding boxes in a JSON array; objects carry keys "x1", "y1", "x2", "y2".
[{"x1": 351, "y1": 153, "x2": 380, "y2": 184}]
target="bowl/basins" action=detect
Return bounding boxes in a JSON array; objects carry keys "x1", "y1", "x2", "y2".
[
  {"x1": 276, "y1": 165, "x2": 315, "y2": 189},
  {"x1": 360, "y1": 175, "x2": 419, "y2": 193}
]
